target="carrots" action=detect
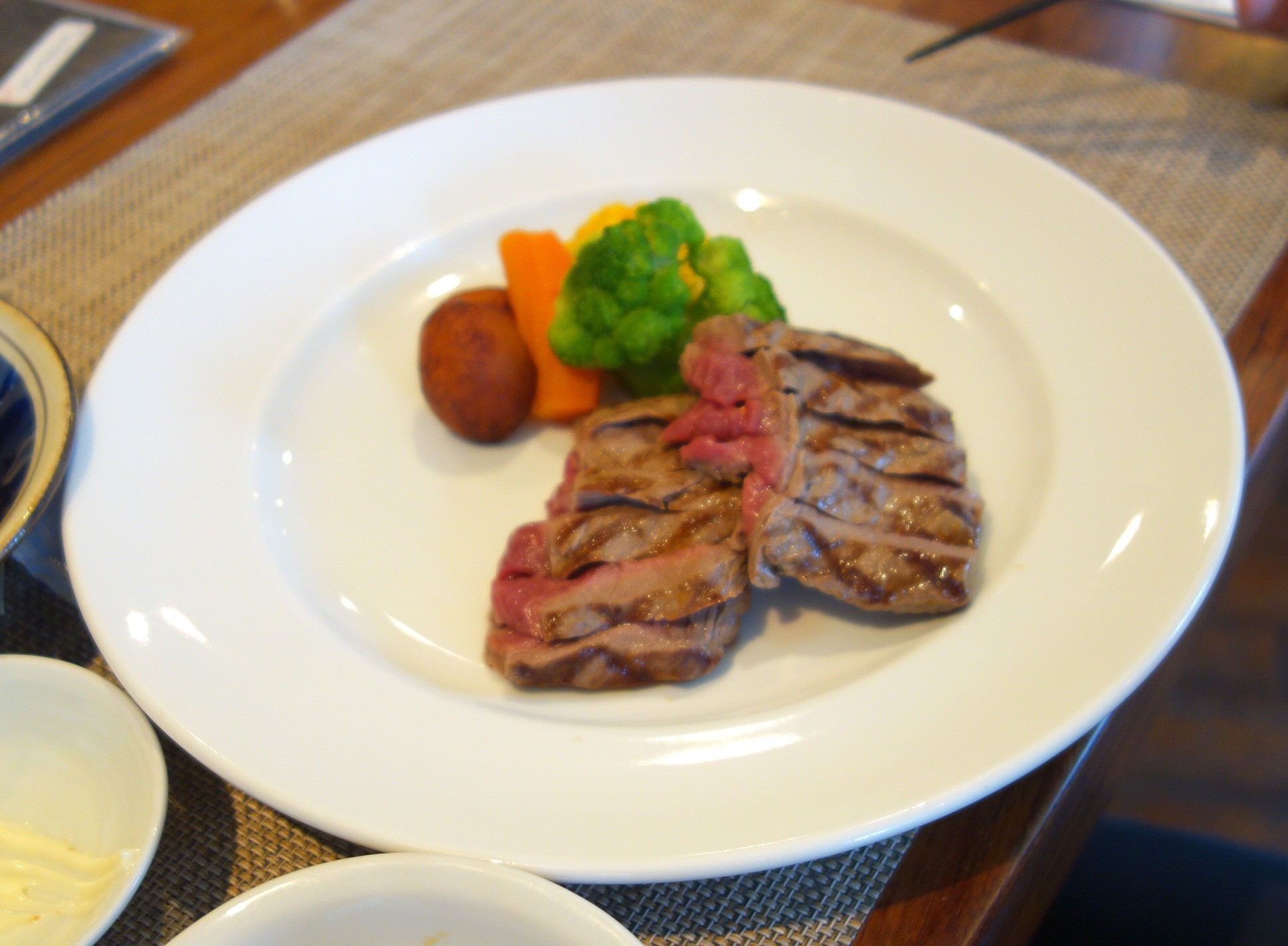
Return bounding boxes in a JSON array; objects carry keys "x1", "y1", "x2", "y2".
[{"x1": 498, "y1": 228, "x2": 605, "y2": 419}]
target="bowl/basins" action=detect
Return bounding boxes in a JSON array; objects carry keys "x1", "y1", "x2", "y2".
[{"x1": 162, "y1": 853, "x2": 641, "y2": 945}]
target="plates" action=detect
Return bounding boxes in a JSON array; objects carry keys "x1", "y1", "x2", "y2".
[
  {"x1": 1, "y1": 652, "x2": 170, "y2": 945},
  {"x1": 0, "y1": 300, "x2": 78, "y2": 556},
  {"x1": 60, "y1": 76, "x2": 1250, "y2": 882}
]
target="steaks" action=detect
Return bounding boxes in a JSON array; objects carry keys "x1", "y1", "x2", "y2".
[
  {"x1": 486, "y1": 398, "x2": 758, "y2": 689},
  {"x1": 662, "y1": 312, "x2": 985, "y2": 620}
]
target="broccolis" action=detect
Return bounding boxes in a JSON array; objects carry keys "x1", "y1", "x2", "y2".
[{"x1": 548, "y1": 197, "x2": 788, "y2": 395}]
